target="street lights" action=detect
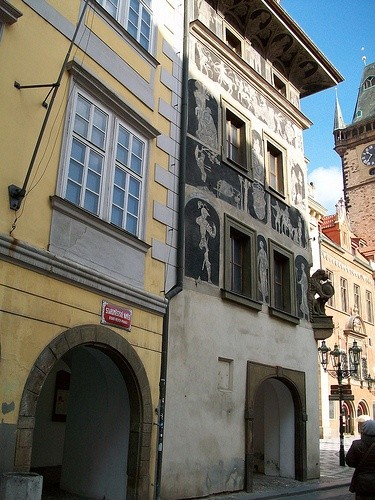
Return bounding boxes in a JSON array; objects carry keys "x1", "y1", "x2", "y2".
[{"x1": 317, "y1": 338, "x2": 362, "y2": 466}]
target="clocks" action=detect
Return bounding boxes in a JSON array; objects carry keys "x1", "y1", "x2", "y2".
[{"x1": 361, "y1": 144, "x2": 375, "y2": 166}]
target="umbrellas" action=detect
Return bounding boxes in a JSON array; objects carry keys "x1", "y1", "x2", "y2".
[{"x1": 355, "y1": 415, "x2": 372, "y2": 422}]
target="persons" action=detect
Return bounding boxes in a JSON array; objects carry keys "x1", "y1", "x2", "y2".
[{"x1": 344, "y1": 420, "x2": 375, "y2": 500}]
